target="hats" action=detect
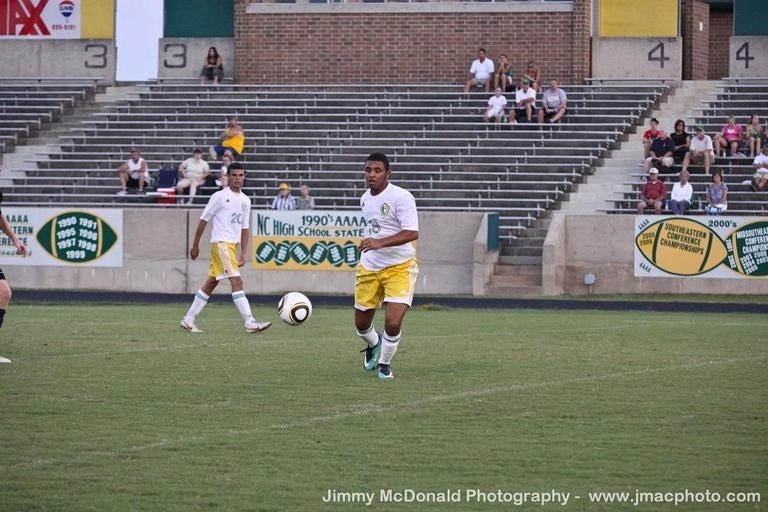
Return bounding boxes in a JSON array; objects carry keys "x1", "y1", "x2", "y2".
[
  {"x1": 278, "y1": 183, "x2": 289, "y2": 191},
  {"x1": 522, "y1": 79, "x2": 531, "y2": 85},
  {"x1": 648, "y1": 167, "x2": 659, "y2": 174}
]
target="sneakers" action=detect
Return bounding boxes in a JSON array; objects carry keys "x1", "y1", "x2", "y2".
[
  {"x1": 376, "y1": 362, "x2": 392, "y2": 380},
  {"x1": 180, "y1": 316, "x2": 202, "y2": 333},
  {"x1": 245, "y1": 319, "x2": 272, "y2": 334},
  {"x1": 359, "y1": 330, "x2": 383, "y2": 371}
]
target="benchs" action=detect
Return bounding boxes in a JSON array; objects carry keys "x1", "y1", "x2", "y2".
[{"x1": 0, "y1": 74, "x2": 767, "y2": 247}]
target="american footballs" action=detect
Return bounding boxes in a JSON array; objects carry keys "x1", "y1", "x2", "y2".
[
  {"x1": 722, "y1": 221, "x2": 768, "y2": 276},
  {"x1": 35, "y1": 213, "x2": 118, "y2": 263},
  {"x1": 636, "y1": 217, "x2": 729, "y2": 276},
  {"x1": 256, "y1": 240, "x2": 360, "y2": 268}
]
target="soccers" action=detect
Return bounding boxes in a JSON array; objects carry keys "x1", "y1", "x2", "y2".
[{"x1": 278, "y1": 292, "x2": 312, "y2": 326}]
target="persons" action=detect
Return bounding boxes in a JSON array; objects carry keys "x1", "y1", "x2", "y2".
[
  {"x1": 198, "y1": 47, "x2": 224, "y2": 86},
  {"x1": 294, "y1": 185, "x2": 315, "y2": 211},
  {"x1": 178, "y1": 163, "x2": 272, "y2": 335},
  {"x1": 173, "y1": 117, "x2": 246, "y2": 203},
  {"x1": 460, "y1": 48, "x2": 567, "y2": 130},
  {"x1": 0, "y1": 186, "x2": 27, "y2": 363},
  {"x1": 354, "y1": 152, "x2": 421, "y2": 379},
  {"x1": 115, "y1": 148, "x2": 150, "y2": 196},
  {"x1": 270, "y1": 182, "x2": 295, "y2": 212},
  {"x1": 637, "y1": 110, "x2": 768, "y2": 216}
]
